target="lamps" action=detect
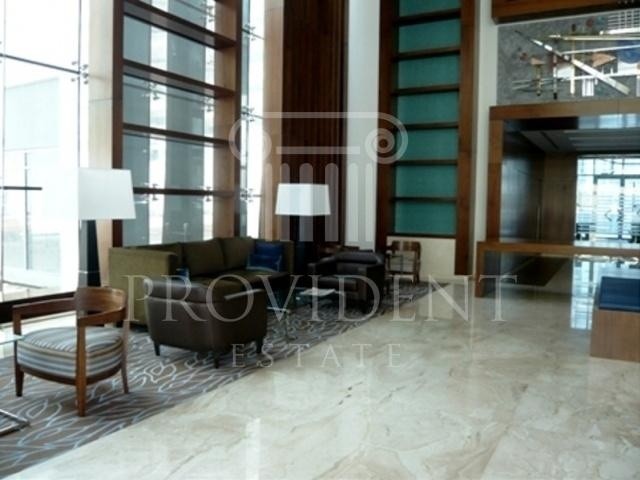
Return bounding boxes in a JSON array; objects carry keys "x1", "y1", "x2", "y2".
[
  {"x1": 275, "y1": 183, "x2": 332, "y2": 217},
  {"x1": 56, "y1": 169, "x2": 136, "y2": 221}
]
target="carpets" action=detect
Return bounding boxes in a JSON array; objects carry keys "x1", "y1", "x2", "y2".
[{"x1": 0, "y1": 281, "x2": 449, "y2": 480}]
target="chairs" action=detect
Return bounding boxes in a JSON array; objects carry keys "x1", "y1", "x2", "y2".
[
  {"x1": 143, "y1": 278, "x2": 268, "y2": 369},
  {"x1": 384, "y1": 240, "x2": 421, "y2": 289},
  {"x1": 306, "y1": 251, "x2": 385, "y2": 313},
  {"x1": 11, "y1": 286, "x2": 130, "y2": 416}
]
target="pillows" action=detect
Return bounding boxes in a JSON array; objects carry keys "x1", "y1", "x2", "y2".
[
  {"x1": 253, "y1": 240, "x2": 285, "y2": 264},
  {"x1": 183, "y1": 238, "x2": 226, "y2": 279},
  {"x1": 134, "y1": 241, "x2": 182, "y2": 276},
  {"x1": 214, "y1": 236, "x2": 254, "y2": 271}
]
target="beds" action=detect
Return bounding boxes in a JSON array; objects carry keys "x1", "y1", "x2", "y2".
[{"x1": 0, "y1": 332, "x2": 30, "y2": 437}]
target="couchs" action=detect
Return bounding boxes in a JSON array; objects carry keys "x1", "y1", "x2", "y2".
[{"x1": 108, "y1": 239, "x2": 296, "y2": 332}]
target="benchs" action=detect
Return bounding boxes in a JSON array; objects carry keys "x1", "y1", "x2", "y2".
[{"x1": 589, "y1": 276, "x2": 639, "y2": 363}]
